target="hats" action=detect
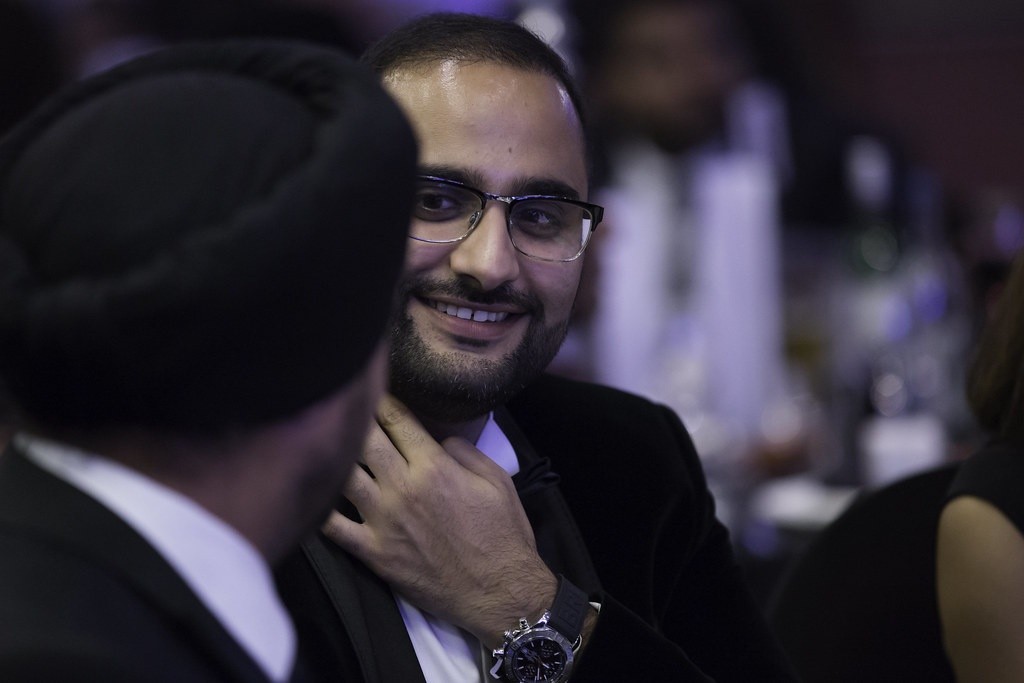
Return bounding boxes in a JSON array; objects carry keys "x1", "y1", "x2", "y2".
[{"x1": 0, "y1": 36, "x2": 419, "y2": 430}]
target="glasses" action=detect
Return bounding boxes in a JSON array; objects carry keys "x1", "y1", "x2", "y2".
[{"x1": 407, "y1": 175, "x2": 604, "y2": 262}]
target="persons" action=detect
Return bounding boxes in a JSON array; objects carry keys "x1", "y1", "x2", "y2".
[
  {"x1": 0, "y1": 28, "x2": 424, "y2": 683},
  {"x1": 561, "y1": 0, "x2": 979, "y2": 569},
  {"x1": 777, "y1": 243, "x2": 1024, "y2": 683},
  {"x1": 260, "y1": 9, "x2": 769, "y2": 683}
]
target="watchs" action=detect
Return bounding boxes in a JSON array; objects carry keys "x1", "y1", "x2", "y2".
[{"x1": 488, "y1": 572, "x2": 592, "y2": 683}]
